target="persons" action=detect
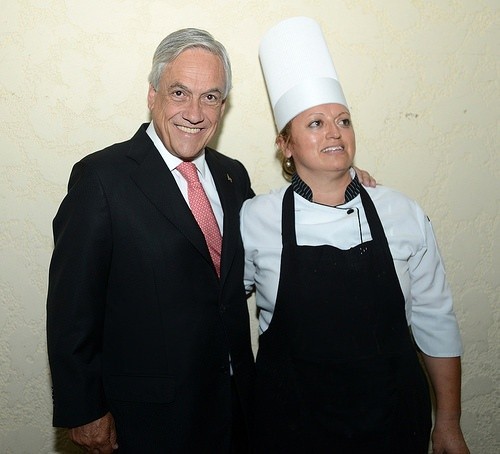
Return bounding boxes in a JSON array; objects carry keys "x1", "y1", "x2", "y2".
[
  {"x1": 47, "y1": 27, "x2": 376, "y2": 454},
  {"x1": 240, "y1": 76, "x2": 471, "y2": 454}
]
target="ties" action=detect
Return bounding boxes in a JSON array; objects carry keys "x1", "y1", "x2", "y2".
[{"x1": 175, "y1": 162, "x2": 222, "y2": 279}]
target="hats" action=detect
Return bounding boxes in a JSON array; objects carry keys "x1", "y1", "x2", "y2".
[{"x1": 258, "y1": 17, "x2": 348, "y2": 132}]
